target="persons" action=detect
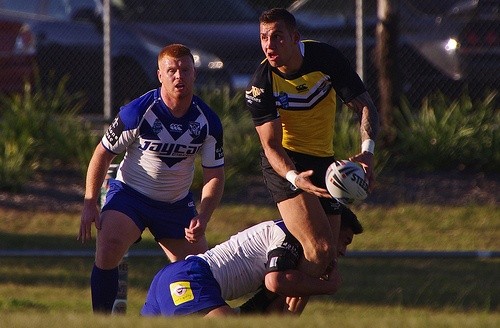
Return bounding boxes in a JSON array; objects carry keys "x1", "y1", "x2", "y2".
[
  {"x1": 140, "y1": 203, "x2": 363, "y2": 318},
  {"x1": 244, "y1": 8, "x2": 379, "y2": 318},
  {"x1": 77, "y1": 44, "x2": 225, "y2": 315}
]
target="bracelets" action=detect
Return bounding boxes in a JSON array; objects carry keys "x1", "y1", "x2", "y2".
[
  {"x1": 361, "y1": 139, "x2": 375, "y2": 155},
  {"x1": 286, "y1": 170, "x2": 300, "y2": 188}
]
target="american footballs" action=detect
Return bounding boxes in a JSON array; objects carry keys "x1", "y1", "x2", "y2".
[{"x1": 325, "y1": 159, "x2": 369, "y2": 207}]
